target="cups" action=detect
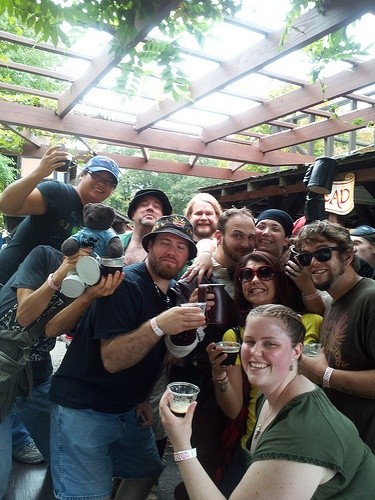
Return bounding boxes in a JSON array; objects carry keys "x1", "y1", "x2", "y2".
[
  {"x1": 302, "y1": 342, "x2": 322, "y2": 358},
  {"x1": 286, "y1": 245, "x2": 300, "y2": 274},
  {"x1": 217, "y1": 342, "x2": 241, "y2": 368},
  {"x1": 166, "y1": 381, "x2": 200, "y2": 415},
  {"x1": 198, "y1": 280, "x2": 225, "y2": 323},
  {"x1": 51, "y1": 151, "x2": 73, "y2": 173},
  {"x1": 182, "y1": 303, "x2": 207, "y2": 320}
]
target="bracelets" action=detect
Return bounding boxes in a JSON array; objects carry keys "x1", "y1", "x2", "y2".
[
  {"x1": 47, "y1": 273, "x2": 60, "y2": 291},
  {"x1": 174, "y1": 446, "x2": 198, "y2": 461},
  {"x1": 211, "y1": 373, "x2": 230, "y2": 392},
  {"x1": 198, "y1": 251, "x2": 213, "y2": 254},
  {"x1": 323, "y1": 367, "x2": 334, "y2": 388},
  {"x1": 303, "y1": 290, "x2": 321, "y2": 301},
  {"x1": 150, "y1": 317, "x2": 164, "y2": 336}
]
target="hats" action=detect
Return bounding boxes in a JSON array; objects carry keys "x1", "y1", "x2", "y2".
[
  {"x1": 349, "y1": 225, "x2": 375, "y2": 245},
  {"x1": 83, "y1": 156, "x2": 119, "y2": 185},
  {"x1": 141, "y1": 215, "x2": 198, "y2": 261},
  {"x1": 127, "y1": 188, "x2": 172, "y2": 222},
  {"x1": 254, "y1": 208, "x2": 293, "y2": 238}
]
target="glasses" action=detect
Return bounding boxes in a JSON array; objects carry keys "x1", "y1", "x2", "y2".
[
  {"x1": 87, "y1": 170, "x2": 115, "y2": 187},
  {"x1": 296, "y1": 247, "x2": 344, "y2": 267},
  {"x1": 238, "y1": 265, "x2": 276, "y2": 284}
]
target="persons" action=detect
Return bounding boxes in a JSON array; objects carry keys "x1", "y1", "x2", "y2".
[{"x1": 0, "y1": 145, "x2": 375, "y2": 500}]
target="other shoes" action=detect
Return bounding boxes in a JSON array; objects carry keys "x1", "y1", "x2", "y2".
[{"x1": 12, "y1": 440, "x2": 44, "y2": 465}]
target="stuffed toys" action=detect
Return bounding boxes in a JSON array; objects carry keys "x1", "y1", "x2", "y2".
[{"x1": 61, "y1": 202, "x2": 123, "y2": 298}]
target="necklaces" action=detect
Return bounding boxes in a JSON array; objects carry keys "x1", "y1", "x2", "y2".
[
  {"x1": 153, "y1": 280, "x2": 162, "y2": 298},
  {"x1": 255, "y1": 375, "x2": 297, "y2": 439}
]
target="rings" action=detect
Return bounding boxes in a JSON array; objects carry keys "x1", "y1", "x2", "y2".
[
  {"x1": 292, "y1": 272, "x2": 297, "y2": 276},
  {"x1": 296, "y1": 267, "x2": 302, "y2": 272},
  {"x1": 209, "y1": 361, "x2": 215, "y2": 364}
]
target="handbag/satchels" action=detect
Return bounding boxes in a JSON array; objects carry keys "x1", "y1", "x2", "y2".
[
  {"x1": 208, "y1": 327, "x2": 251, "y2": 488},
  {"x1": 0, "y1": 330, "x2": 36, "y2": 424}
]
